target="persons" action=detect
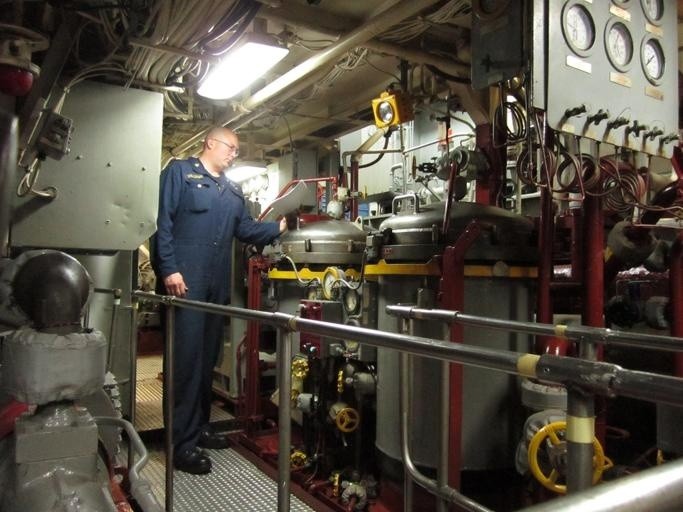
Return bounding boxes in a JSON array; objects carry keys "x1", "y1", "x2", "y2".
[{"x1": 148, "y1": 125, "x2": 289, "y2": 475}]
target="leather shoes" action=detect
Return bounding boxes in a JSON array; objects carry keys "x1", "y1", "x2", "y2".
[
  {"x1": 174, "y1": 449, "x2": 211, "y2": 474},
  {"x1": 198, "y1": 429, "x2": 229, "y2": 449}
]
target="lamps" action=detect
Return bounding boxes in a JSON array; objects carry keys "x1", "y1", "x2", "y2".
[{"x1": 196, "y1": 32, "x2": 290, "y2": 101}]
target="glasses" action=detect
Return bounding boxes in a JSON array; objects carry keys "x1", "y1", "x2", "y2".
[{"x1": 213, "y1": 139, "x2": 239, "y2": 156}]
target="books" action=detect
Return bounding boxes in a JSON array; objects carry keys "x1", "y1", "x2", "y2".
[{"x1": 269, "y1": 180, "x2": 311, "y2": 217}]
